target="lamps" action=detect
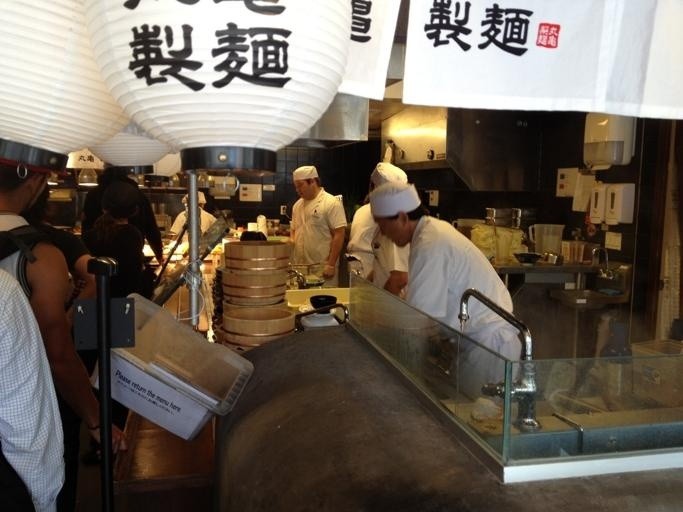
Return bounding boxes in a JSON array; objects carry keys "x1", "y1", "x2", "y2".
[
  {"x1": 46, "y1": 174, "x2": 59, "y2": 186},
  {"x1": 75, "y1": 167, "x2": 99, "y2": 187}
]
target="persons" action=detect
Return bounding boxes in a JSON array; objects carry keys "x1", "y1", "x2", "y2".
[
  {"x1": 21, "y1": 159, "x2": 166, "y2": 464},
  {"x1": 167, "y1": 188, "x2": 216, "y2": 242},
  {"x1": 0, "y1": 162, "x2": 130, "y2": 510},
  {"x1": 344, "y1": 222, "x2": 410, "y2": 297},
  {"x1": 367, "y1": 181, "x2": 525, "y2": 403},
  {"x1": 0, "y1": 268, "x2": 67, "y2": 508},
  {"x1": 349, "y1": 162, "x2": 408, "y2": 237},
  {"x1": 279, "y1": 165, "x2": 347, "y2": 288}
]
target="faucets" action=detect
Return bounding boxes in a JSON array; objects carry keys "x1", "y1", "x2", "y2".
[
  {"x1": 591, "y1": 247, "x2": 609, "y2": 275},
  {"x1": 458, "y1": 289, "x2": 543, "y2": 435}
]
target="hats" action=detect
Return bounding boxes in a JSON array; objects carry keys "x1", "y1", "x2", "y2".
[
  {"x1": 370, "y1": 182, "x2": 421, "y2": 217},
  {"x1": 182, "y1": 191, "x2": 207, "y2": 204},
  {"x1": 293, "y1": 166, "x2": 319, "y2": 182},
  {"x1": 371, "y1": 162, "x2": 408, "y2": 186}
]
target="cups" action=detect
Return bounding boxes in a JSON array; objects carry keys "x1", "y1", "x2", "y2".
[{"x1": 528, "y1": 223, "x2": 565, "y2": 256}]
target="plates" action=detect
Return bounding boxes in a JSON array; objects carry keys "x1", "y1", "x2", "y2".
[{"x1": 297, "y1": 277, "x2": 325, "y2": 287}]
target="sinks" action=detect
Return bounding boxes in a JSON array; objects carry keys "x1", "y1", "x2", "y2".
[{"x1": 553, "y1": 290, "x2": 606, "y2": 312}]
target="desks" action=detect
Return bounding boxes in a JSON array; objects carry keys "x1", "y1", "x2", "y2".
[{"x1": 487, "y1": 264, "x2": 601, "y2": 384}]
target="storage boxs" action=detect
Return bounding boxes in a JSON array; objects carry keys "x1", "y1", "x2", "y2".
[
  {"x1": 627, "y1": 339, "x2": 683, "y2": 408},
  {"x1": 87, "y1": 291, "x2": 254, "y2": 446}
]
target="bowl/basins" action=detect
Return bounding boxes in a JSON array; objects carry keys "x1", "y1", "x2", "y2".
[
  {"x1": 309, "y1": 294, "x2": 336, "y2": 313},
  {"x1": 218, "y1": 240, "x2": 297, "y2": 351},
  {"x1": 511, "y1": 252, "x2": 540, "y2": 264}
]
